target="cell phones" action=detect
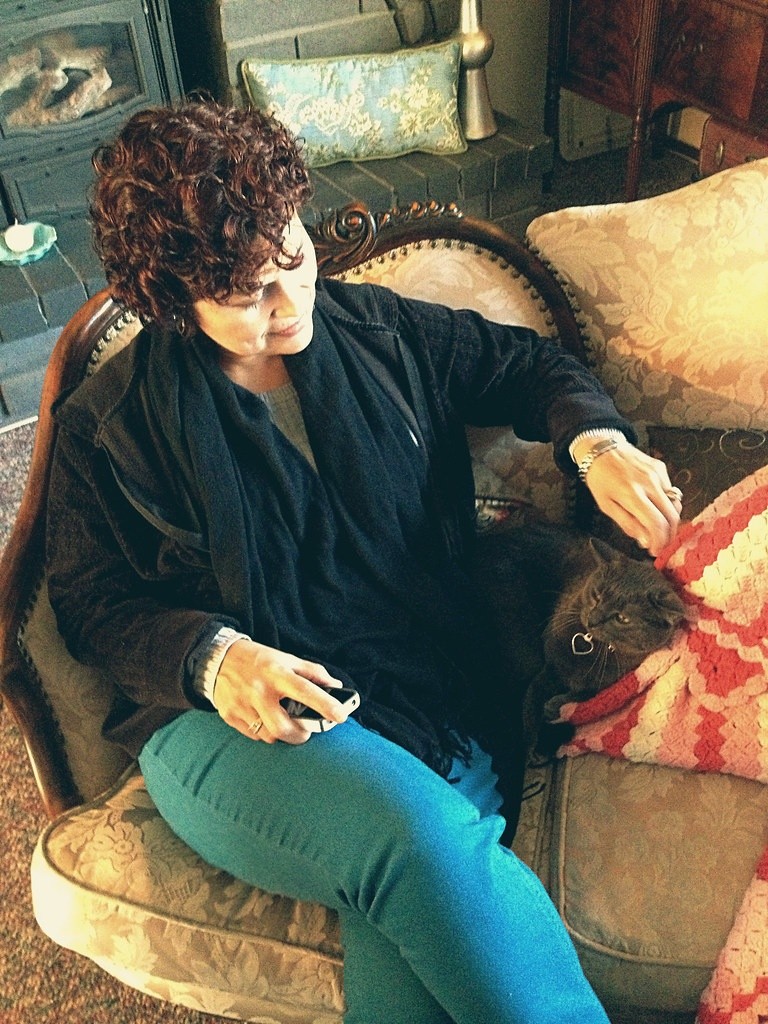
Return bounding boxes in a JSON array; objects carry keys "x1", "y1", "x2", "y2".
[{"x1": 278, "y1": 686, "x2": 361, "y2": 733}]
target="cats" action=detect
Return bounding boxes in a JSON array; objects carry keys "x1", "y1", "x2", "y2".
[{"x1": 473, "y1": 523, "x2": 685, "y2": 733}]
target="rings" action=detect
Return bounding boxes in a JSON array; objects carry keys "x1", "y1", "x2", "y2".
[{"x1": 247, "y1": 717, "x2": 263, "y2": 735}]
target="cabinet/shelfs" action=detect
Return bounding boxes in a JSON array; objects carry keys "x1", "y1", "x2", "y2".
[{"x1": 540, "y1": 0, "x2": 766, "y2": 203}]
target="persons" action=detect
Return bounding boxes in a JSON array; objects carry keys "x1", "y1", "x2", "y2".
[{"x1": 44, "y1": 106, "x2": 684, "y2": 1022}]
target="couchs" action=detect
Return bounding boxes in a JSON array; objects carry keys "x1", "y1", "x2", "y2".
[{"x1": 2, "y1": 201, "x2": 768, "y2": 1022}]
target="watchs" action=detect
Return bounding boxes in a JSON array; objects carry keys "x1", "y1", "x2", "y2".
[{"x1": 579, "y1": 437, "x2": 625, "y2": 479}]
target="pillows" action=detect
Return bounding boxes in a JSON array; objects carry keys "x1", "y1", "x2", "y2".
[
  {"x1": 241, "y1": 38, "x2": 468, "y2": 169},
  {"x1": 527, "y1": 152, "x2": 768, "y2": 436}
]
